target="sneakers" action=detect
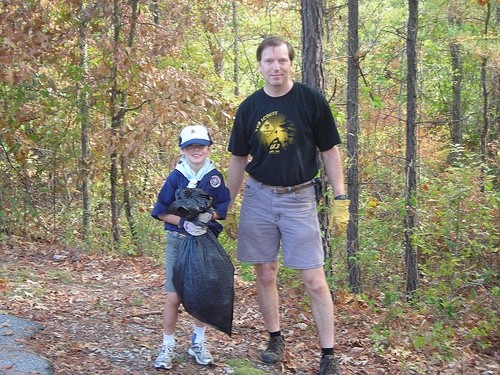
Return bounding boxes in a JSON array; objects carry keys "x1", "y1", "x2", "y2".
[
  {"x1": 188, "y1": 342, "x2": 214, "y2": 365},
  {"x1": 260, "y1": 335, "x2": 286, "y2": 363},
  {"x1": 154, "y1": 344, "x2": 177, "y2": 370},
  {"x1": 319, "y1": 355, "x2": 341, "y2": 375}
]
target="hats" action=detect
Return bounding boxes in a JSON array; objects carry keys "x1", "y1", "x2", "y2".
[{"x1": 179, "y1": 126, "x2": 213, "y2": 148}]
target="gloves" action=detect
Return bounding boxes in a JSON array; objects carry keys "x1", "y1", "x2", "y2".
[
  {"x1": 224, "y1": 211, "x2": 239, "y2": 240},
  {"x1": 179, "y1": 210, "x2": 213, "y2": 236},
  {"x1": 326, "y1": 198, "x2": 351, "y2": 234}
]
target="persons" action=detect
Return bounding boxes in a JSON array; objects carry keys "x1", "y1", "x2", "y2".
[
  {"x1": 151, "y1": 125, "x2": 231, "y2": 370},
  {"x1": 227, "y1": 39, "x2": 350, "y2": 375}
]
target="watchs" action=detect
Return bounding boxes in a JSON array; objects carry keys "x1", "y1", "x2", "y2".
[{"x1": 334, "y1": 194, "x2": 346, "y2": 200}]
